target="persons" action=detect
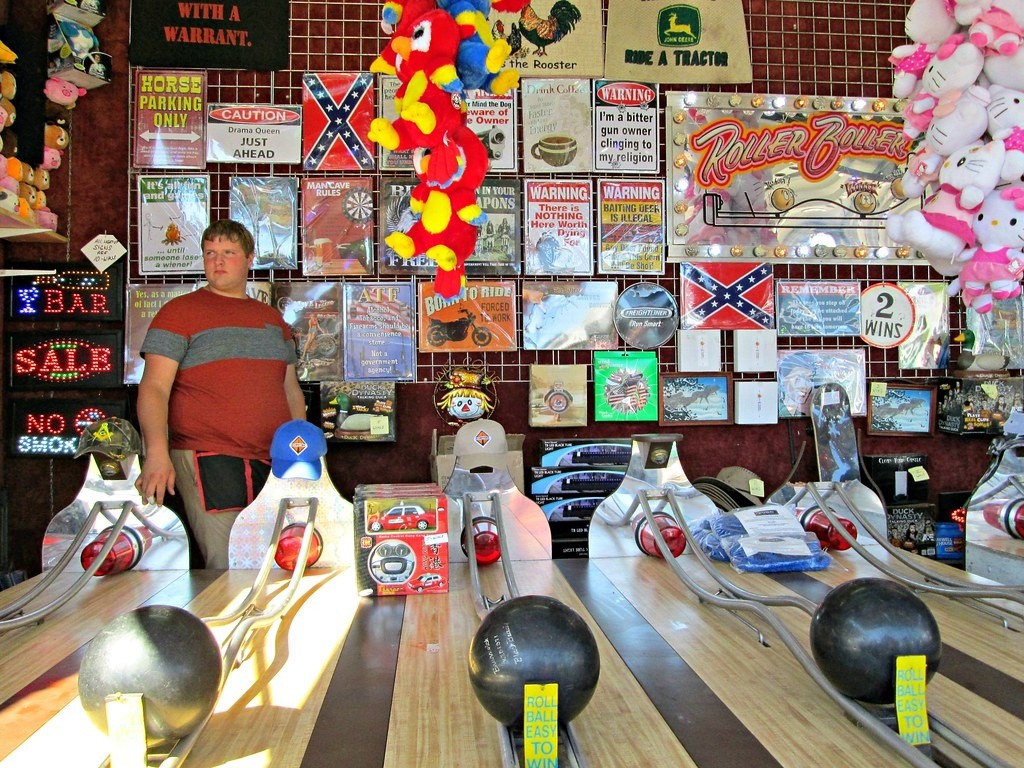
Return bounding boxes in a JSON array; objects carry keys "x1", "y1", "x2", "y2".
[
  {"x1": 778, "y1": 351, "x2": 817, "y2": 417},
  {"x1": 474, "y1": 223, "x2": 484, "y2": 257},
  {"x1": 89, "y1": 54, "x2": 107, "y2": 79},
  {"x1": 536, "y1": 232, "x2": 561, "y2": 273},
  {"x1": 938, "y1": 378, "x2": 1024, "y2": 435},
  {"x1": 301, "y1": 313, "x2": 325, "y2": 360},
  {"x1": 498, "y1": 218, "x2": 512, "y2": 252},
  {"x1": 485, "y1": 220, "x2": 496, "y2": 252},
  {"x1": 136, "y1": 218, "x2": 307, "y2": 569},
  {"x1": 887, "y1": 519, "x2": 935, "y2": 548}
]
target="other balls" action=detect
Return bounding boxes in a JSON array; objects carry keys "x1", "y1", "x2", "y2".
[
  {"x1": 469, "y1": 596, "x2": 600, "y2": 730},
  {"x1": 810, "y1": 578, "x2": 943, "y2": 703},
  {"x1": 78, "y1": 605, "x2": 222, "y2": 740}
]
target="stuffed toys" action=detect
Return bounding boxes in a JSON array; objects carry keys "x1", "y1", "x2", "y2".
[
  {"x1": 0, "y1": 38, "x2": 87, "y2": 233},
  {"x1": 886, "y1": 0, "x2": 1023, "y2": 312},
  {"x1": 368, "y1": 0, "x2": 521, "y2": 299}
]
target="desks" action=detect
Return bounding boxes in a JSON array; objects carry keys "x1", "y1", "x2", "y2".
[{"x1": 1, "y1": 538, "x2": 1023, "y2": 765}]
